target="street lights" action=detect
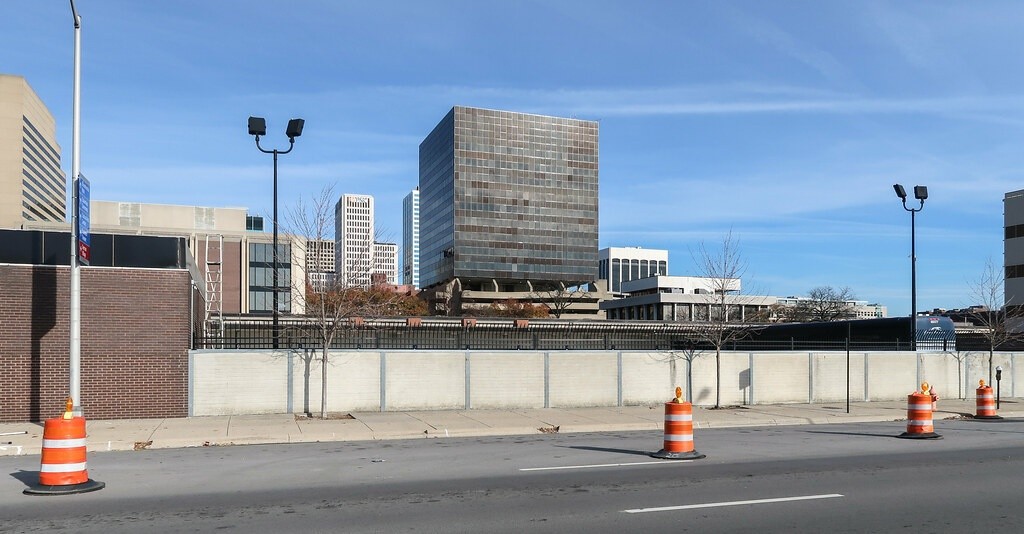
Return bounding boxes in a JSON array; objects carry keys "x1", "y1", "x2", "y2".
[
  {"x1": 247, "y1": 116, "x2": 305, "y2": 350},
  {"x1": 893, "y1": 183, "x2": 929, "y2": 351}
]
